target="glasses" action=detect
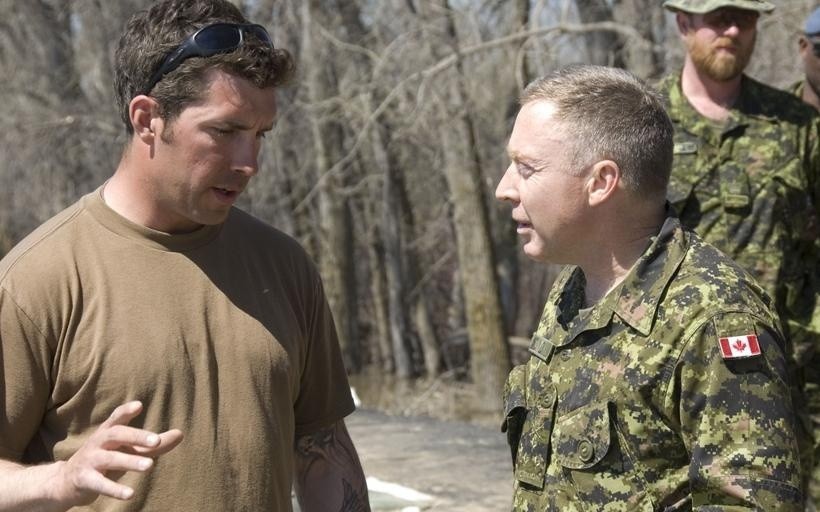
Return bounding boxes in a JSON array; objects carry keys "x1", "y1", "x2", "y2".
[
  {"x1": 808, "y1": 39, "x2": 820, "y2": 50},
  {"x1": 142, "y1": 18, "x2": 275, "y2": 95}
]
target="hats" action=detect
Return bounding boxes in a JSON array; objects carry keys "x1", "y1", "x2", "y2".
[
  {"x1": 661, "y1": 0, "x2": 779, "y2": 16},
  {"x1": 801, "y1": 5, "x2": 820, "y2": 36}
]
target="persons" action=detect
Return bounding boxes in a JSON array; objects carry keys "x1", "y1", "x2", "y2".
[
  {"x1": 638, "y1": 0, "x2": 818, "y2": 510},
  {"x1": 496, "y1": 65, "x2": 818, "y2": 511},
  {"x1": 779, "y1": 14, "x2": 818, "y2": 111},
  {"x1": 0, "y1": 2, "x2": 371, "y2": 510}
]
